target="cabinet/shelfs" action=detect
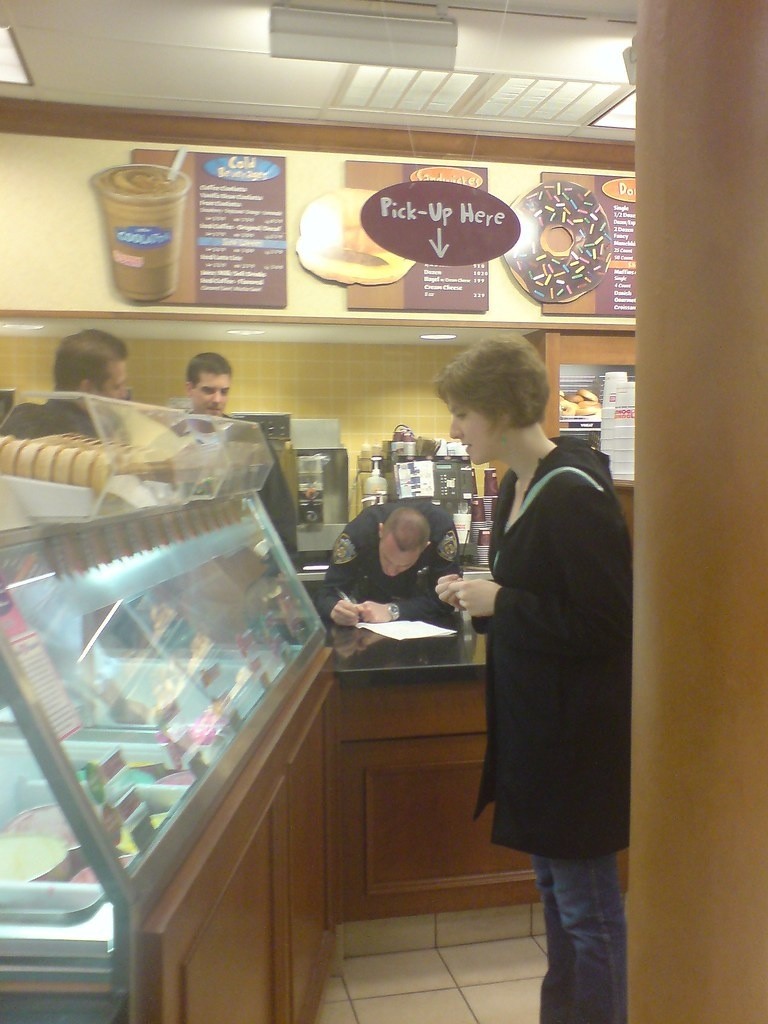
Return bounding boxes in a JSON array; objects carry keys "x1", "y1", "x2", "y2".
[{"x1": 135, "y1": 650, "x2": 627, "y2": 1024}]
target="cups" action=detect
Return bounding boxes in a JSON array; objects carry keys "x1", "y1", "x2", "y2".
[
  {"x1": 389, "y1": 426, "x2": 466, "y2": 459},
  {"x1": 451, "y1": 468, "x2": 499, "y2": 566},
  {"x1": 600, "y1": 372, "x2": 635, "y2": 481},
  {"x1": 90, "y1": 164, "x2": 191, "y2": 300}
]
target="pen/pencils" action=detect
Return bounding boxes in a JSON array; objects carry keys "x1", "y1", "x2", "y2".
[{"x1": 335, "y1": 587, "x2": 353, "y2": 605}]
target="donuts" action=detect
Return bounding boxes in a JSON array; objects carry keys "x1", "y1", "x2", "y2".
[{"x1": 500, "y1": 180, "x2": 614, "y2": 304}]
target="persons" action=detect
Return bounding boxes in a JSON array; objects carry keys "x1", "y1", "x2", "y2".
[
  {"x1": 3, "y1": 327, "x2": 302, "y2": 651},
  {"x1": 318, "y1": 501, "x2": 462, "y2": 680},
  {"x1": 435, "y1": 332, "x2": 633, "y2": 1024}
]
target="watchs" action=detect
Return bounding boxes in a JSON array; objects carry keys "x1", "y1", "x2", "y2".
[{"x1": 389, "y1": 601, "x2": 401, "y2": 621}]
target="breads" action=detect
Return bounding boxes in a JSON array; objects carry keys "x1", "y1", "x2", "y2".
[
  {"x1": 296, "y1": 187, "x2": 419, "y2": 285},
  {"x1": 559, "y1": 389, "x2": 601, "y2": 418}
]
